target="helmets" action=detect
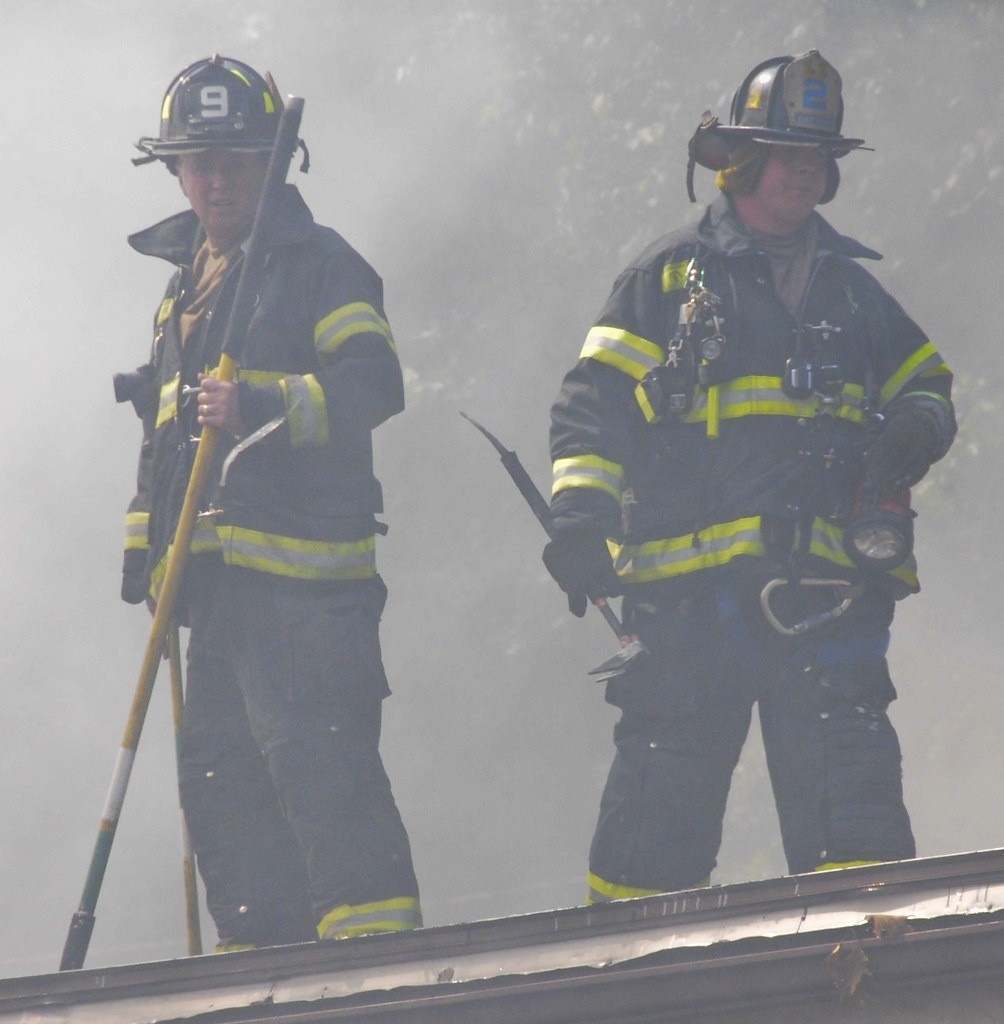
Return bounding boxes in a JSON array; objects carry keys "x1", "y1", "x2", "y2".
[
  {"x1": 134, "y1": 53, "x2": 297, "y2": 157},
  {"x1": 696, "y1": 47, "x2": 864, "y2": 157}
]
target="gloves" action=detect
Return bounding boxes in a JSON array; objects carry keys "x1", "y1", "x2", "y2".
[
  {"x1": 548, "y1": 518, "x2": 624, "y2": 619},
  {"x1": 862, "y1": 405, "x2": 940, "y2": 493}
]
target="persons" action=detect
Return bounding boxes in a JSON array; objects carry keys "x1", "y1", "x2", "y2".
[
  {"x1": 541, "y1": 45, "x2": 959, "y2": 909},
  {"x1": 114, "y1": 51, "x2": 424, "y2": 957}
]
